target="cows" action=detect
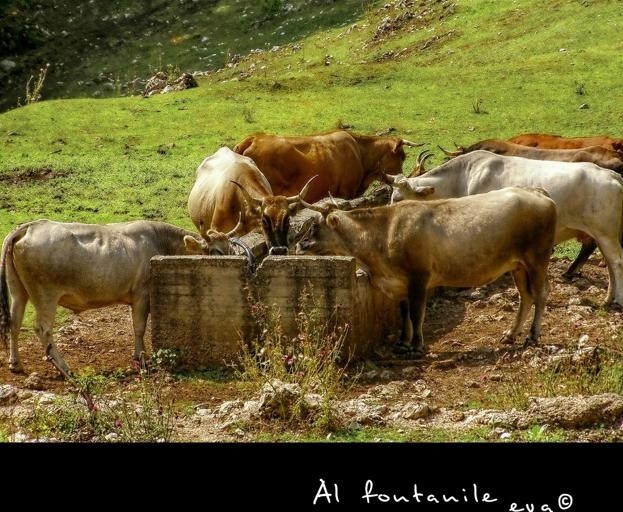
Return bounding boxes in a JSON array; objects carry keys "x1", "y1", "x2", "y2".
[
  {"x1": 377, "y1": 133, "x2": 623, "y2": 314},
  {"x1": 295, "y1": 186, "x2": 557, "y2": 360},
  {"x1": 233, "y1": 129, "x2": 425, "y2": 210},
  {"x1": 0, "y1": 211, "x2": 243, "y2": 380},
  {"x1": 188, "y1": 146, "x2": 319, "y2": 255}
]
